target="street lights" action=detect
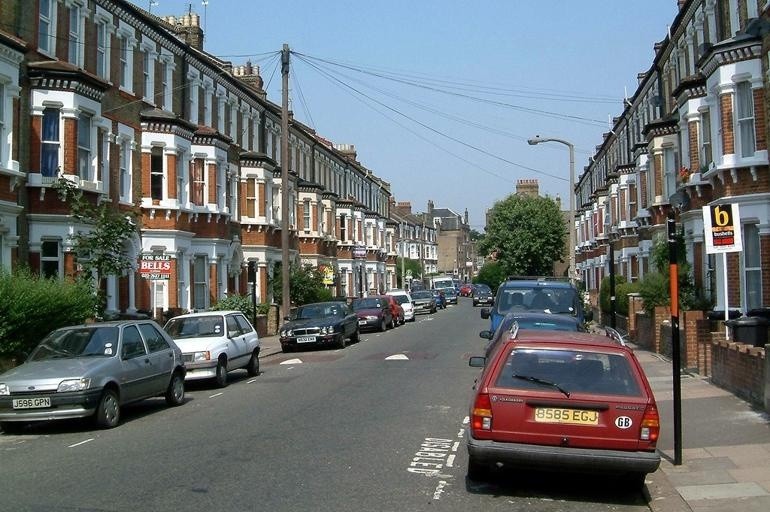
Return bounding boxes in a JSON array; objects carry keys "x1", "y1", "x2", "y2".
[
  {"x1": 402, "y1": 213, "x2": 423, "y2": 290},
  {"x1": 527, "y1": 136, "x2": 578, "y2": 291}
]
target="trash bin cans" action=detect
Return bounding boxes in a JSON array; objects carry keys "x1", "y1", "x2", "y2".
[{"x1": 721, "y1": 317, "x2": 770, "y2": 348}]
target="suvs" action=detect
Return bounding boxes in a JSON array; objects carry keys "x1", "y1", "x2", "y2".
[{"x1": 481, "y1": 274, "x2": 586, "y2": 337}]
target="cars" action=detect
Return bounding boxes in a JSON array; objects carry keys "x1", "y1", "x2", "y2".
[
  {"x1": 153, "y1": 310, "x2": 260, "y2": 387},
  {"x1": 370, "y1": 293, "x2": 405, "y2": 326},
  {"x1": 0, "y1": 319, "x2": 187, "y2": 429},
  {"x1": 345, "y1": 297, "x2": 394, "y2": 333},
  {"x1": 478, "y1": 313, "x2": 588, "y2": 359},
  {"x1": 384, "y1": 290, "x2": 415, "y2": 322},
  {"x1": 467, "y1": 320, "x2": 661, "y2": 495},
  {"x1": 409, "y1": 276, "x2": 494, "y2": 315},
  {"x1": 279, "y1": 302, "x2": 361, "y2": 352}
]
